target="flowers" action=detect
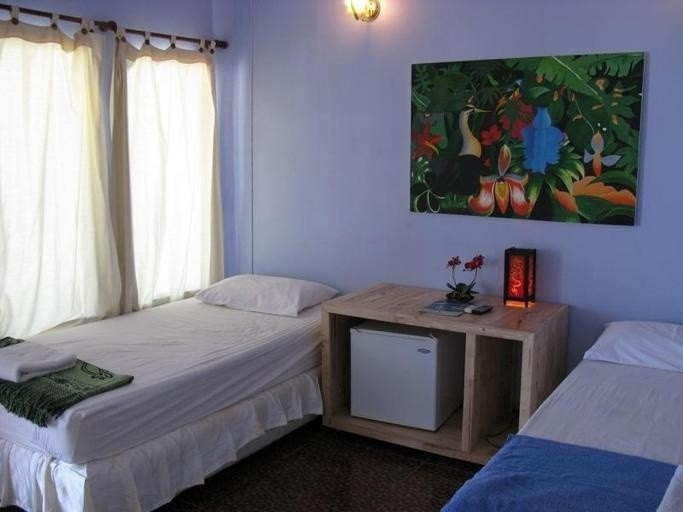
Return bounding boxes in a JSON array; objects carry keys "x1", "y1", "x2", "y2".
[{"x1": 445, "y1": 254, "x2": 483, "y2": 300}]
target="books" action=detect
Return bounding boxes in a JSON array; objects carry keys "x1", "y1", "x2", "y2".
[{"x1": 417, "y1": 299, "x2": 474, "y2": 317}]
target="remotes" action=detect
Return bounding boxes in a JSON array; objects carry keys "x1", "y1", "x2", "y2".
[
  {"x1": 464, "y1": 303, "x2": 480, "y2": 313},
  {"x1": 473, "y1": 304, "x2": 494, "y2": 313}
]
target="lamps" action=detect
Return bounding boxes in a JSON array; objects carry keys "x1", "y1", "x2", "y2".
[
  {"x1": 341, "y1": 0, "x2": 380, "y2": 21},
  {"x1": 502, "y1": 246, "x2": 536, "y2": 307}
]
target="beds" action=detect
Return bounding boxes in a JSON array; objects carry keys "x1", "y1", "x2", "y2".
[
  {"x1": 435, "y1": 348, "x2": 682, "y2": 510},
  {"x1": 0, "y1": 296, "x2": 320, "y2": 512}
]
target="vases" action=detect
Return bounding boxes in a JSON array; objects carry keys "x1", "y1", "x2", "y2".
[{"x1": 446, "y1": 291, "x2": 474, "y2": 304}]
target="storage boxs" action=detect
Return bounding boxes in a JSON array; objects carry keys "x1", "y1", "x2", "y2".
[{"x1": 346, "y1": 323, "x2": 464, "y2": 432}]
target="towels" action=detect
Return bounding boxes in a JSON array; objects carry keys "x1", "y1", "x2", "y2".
[{"x1": 0, "y1": 340, "x2": 75, "y2": 382}]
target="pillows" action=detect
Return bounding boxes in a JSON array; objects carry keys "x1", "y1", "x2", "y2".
[
  {"x1": 581, "y1": 318, "x2": 683, "y2": 372},
  {"x1": 195, "y1": 273, "x2": 344, "y2": 321}
]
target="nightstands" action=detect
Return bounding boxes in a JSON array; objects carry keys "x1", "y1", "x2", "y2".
[{"x1": 318, "y1": 283, "x2": 569, "y2": 468}]
